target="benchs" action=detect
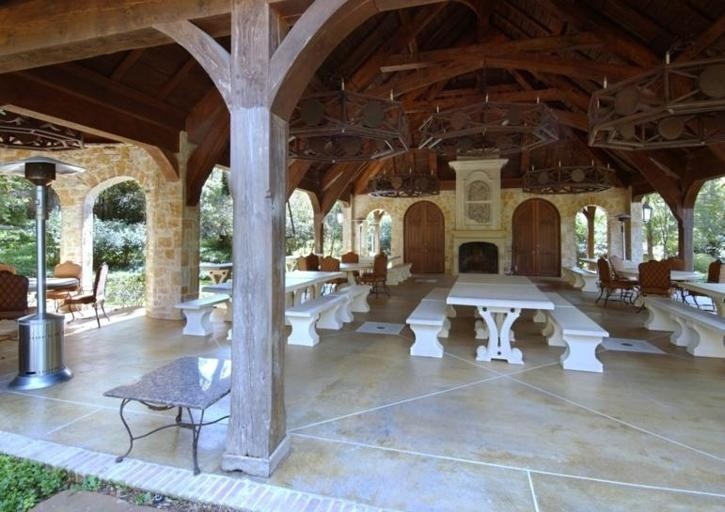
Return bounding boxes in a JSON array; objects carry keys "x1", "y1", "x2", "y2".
[
  {"x1": 175, "y1": 295, "x2": 232, "y2": 341},
  {"x1": 424, "y1": 288, "x2": 456, "y2": 330},
  {"x1": 406, "y1": 301, "x2": 451, "y2": 358},
  {"x1": 547, "y1": 307, "x2": 609, "y2": 373},
  {"x1": 533, "y1": 292, "x2": 580, "y2": 336},
  {"x1": 385, "y1": 262, "x2": 413, "y2": 287},
  {"x1": 642, "y1": 297, "x2": 725, "y2": 358},
  {"x1": 564, "y1": 264, "x2": 598, "y2": 292},
  {"x1": 282, "y1": 284, "x2": 370, "y2": 345}
]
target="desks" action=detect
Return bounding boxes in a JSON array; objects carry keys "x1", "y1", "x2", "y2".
[
  {"x1": 579, "y1": 258, "x2": 598, "y2": 270},
  {"x1": 202, "y1": 270, "x2": 344, "y2": 307},
  {"x1": 448, "y1": 273, "x2": 557, "y2": 364},
  {"x1": 679, "y1": 277, "x2": 725, "y2": 320},
  {"x1": 99, "y1": 352, "x2": 233, "y2": 476}
]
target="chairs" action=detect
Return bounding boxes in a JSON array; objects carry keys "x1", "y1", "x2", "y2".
[
  {"x1": 297, "y1": 252, "x2": 391, "y2": 298},
  {"x1": 0, "y1": 260, "x2": 111, "y2": 328},
  {"x1": 596, "y1": 254, "x2": 725, "y2": 312}
]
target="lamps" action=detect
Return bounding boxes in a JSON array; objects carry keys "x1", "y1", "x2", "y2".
[
  {"x1": 416, "y1": 85, "x2": 563, "y2": 160},
  {"x1": 583, "y1": 46, "x2": 725, "y2": 153},
  {"x1": 286, "y1": 64, "x2": 411, "y2": 168},
  {"x1": 642, "y1": 202, "x2": 653, "y2": 224},
  {"x1": 0, "y1": 109, "x2": 85, "y2": 156},
  {"x1": 522, "y1": 152, "x2": 618, "y2": 197},
  {"x1": 366, "y1": 160, "x2": 443, "y2": 200}
]
